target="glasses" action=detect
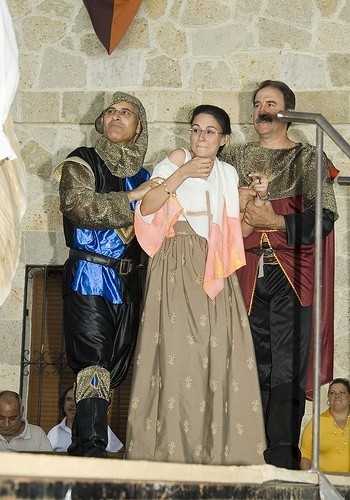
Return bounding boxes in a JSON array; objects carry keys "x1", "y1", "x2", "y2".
[
  {"x1": 105, "y1": 109, "x2": 138, "y2": 120},
  {"x1": 189, "y1": 128, "x2": 223, "y2": 137}
]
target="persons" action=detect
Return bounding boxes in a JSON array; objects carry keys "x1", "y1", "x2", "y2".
[
  {"x1": 219, "y1": 80, "x2": 340, "y2": 471},
  {"x1": 135, "y1": 104, "x2": 267, "y2": 465},
  {"x1": 53, "y1": 91, "x2": 166, "y2": 457},
  {"x1": 47, "y1": 387, "x2": 125, "y2": 453},
  {"x1": 300, "y1": 378, "x2": 350, "y2": 472},
  {"x1": 0, "y1": 391, "x2": 54, "y2": 451}
]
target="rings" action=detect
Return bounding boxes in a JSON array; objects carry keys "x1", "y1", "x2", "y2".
[{"x1": 259, "y1": 179, "x2": 261, "y2": 184}]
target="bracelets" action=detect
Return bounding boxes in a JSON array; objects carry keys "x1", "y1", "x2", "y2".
[
  {"x1": 257, "y1": 193, "x2": 269, "y2": 200},
  {"x1": 163, "y1": 183, "x2": 176, "y2": 197}
]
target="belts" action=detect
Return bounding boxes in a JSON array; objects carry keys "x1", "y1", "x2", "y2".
[{"x1": 68, "y1": 249, "x2": 136, "y2": 275}]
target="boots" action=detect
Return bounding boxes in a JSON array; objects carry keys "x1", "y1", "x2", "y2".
[{"x1": 74, "y1": 365, "x2": 110, "y2": 458}]
